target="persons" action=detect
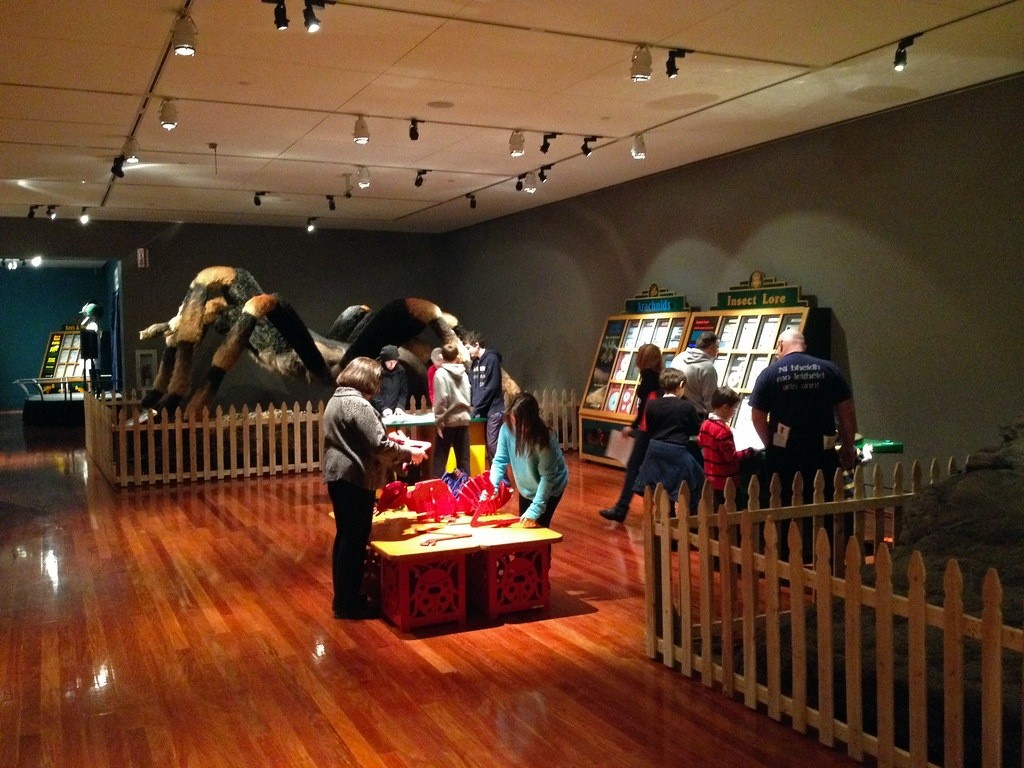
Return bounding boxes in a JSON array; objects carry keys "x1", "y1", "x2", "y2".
[
  {"x1": 432, "y1": 344, "x2": 471, "y2": 481},
  {"x1": 632, "y1": 368, "x2": 702, "y2": 552},
  {"x1": 427, "y1": 347, "x2": 446, "y2": 414},
  {"x1": 598, "y1": 344, "x2": 667, "y2": 524},
  {"x1": 670, "y1": 330, "x2": 719, "y2": 472},
  {"x1": 748, "y1": 327, "x2": 856, "y2": 587},
  {"x1": 366, "y1": 345, "x2": 409, "y2": 418},
  {"x1": 478, "y1": 392, "x2": 569, "y2": 602},
  {"x1": 461, "y1": 330, "x2": 512, "y2": 487},
  {"x1": 321, "y1": 357, "x2": 429, "y2": 619},
  {"x1": 696, "y1": 386, "x2": 764, "y2": 572}
]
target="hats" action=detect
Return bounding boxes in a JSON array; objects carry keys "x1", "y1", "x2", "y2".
[{"x1": 380, "y1": 345, "x2": 398, "y2": 361}]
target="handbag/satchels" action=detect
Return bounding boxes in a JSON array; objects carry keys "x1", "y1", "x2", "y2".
[{"x1": 442, "y1": 467, "x2": 468, "y2": 498}]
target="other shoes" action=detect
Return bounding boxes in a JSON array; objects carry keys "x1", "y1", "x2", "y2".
[
  {"x1": 333, "y1": 594, "x2": 368, "y2": 620},
  {"x1": 504, "y1": 478, "x2": 511, "y2": 486},
  {"x1": 600, "y1": 509, "x2": 625, "y2": 522}
]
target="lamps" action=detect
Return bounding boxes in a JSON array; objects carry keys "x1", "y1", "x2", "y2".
[
  {"x1": 893, "y1": 37, "x2": 914, "y2": 72},
  {"x1": 415, "y1": 170, "x2": 427, "y2": 187},
  {"x1": 28, "y1": 205, "x2": 89, "y2": 225},
  {"x1": 353, "y1": 116, "x2": 369, "y2": 145},
  {"x1": 305, "y1": 165, "x2": 372, "y2": 232},
  {"x1": 630, "y1": 44, "x2": 685, "y2": 82},
  {"x1": 111, "y1": 136, "x2": 141, "y2": 178},
  {"x1": 409, "y1": 119, "x2": 419, "y2": 140},
  {"x1": 169, "y1": 8, "x2": 199, "y2": 57},
  {"x1": 466, "y1": 193, "x2": 476, "y2": 208},
  {"x1": 631, "y1": 133, "x2": 647, "y2": 160},
  {"x1": 262, "y1": 0, "x2": 325, "y2": 33},
  {"x1": 516, "y1": 165, "x2": 551, "y2": 193},
  {"x1": 508, "y1": 129, "x2": 556, "y2": 158},
  {"x1": 158, "y1": 96, "x2": 179, "y2": 131},
  {"x1": 581, "y1": 136, "x2": 598, "y2": 158},
  {"x1": 254, "y1": 191, "x2": 265, "y2": 206}
]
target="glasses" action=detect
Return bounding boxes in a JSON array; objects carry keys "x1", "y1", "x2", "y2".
[{"x1": 774, "y1": 341, "x2": 783, "y2": 355}]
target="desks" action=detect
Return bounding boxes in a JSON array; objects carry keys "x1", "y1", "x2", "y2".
[{"x1": 328, "y1": 485, "x2": 562, "y2": 633}]
